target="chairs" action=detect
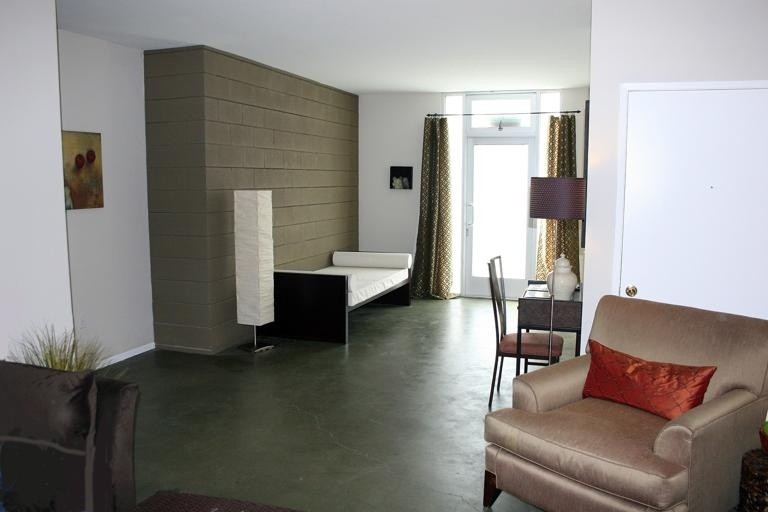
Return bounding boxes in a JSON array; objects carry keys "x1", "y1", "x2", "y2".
[
  {"x1": 487, "y1": 254, "x2": 565, "y2": 412},
  {"x1": 482, "y1": 294, "x2": 768, "y2": 511}
]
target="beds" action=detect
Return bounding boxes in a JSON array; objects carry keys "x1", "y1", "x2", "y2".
[{"x1": 275, "y1": 252, "x2": 413, "y2": 346}]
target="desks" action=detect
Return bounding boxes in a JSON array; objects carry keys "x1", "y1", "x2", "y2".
[{"x1": 516, "y1": 280, "x2": 583, "y2": 375}]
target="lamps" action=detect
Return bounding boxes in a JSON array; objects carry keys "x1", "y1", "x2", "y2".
[
  {"x1": 233, "y1": 189, "x2": 275, "y2": 354},
  {"x1": 529, "y1": 176, "x2": 586, "y2": 365}
]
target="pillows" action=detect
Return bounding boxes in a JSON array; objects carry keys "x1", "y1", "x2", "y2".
[
  {"x1": 332, "y1": 251, "x2": 413, "y2": 270},
  {"x1": 582, "y1": 338, "x2": 718, "y2": 422}
]
